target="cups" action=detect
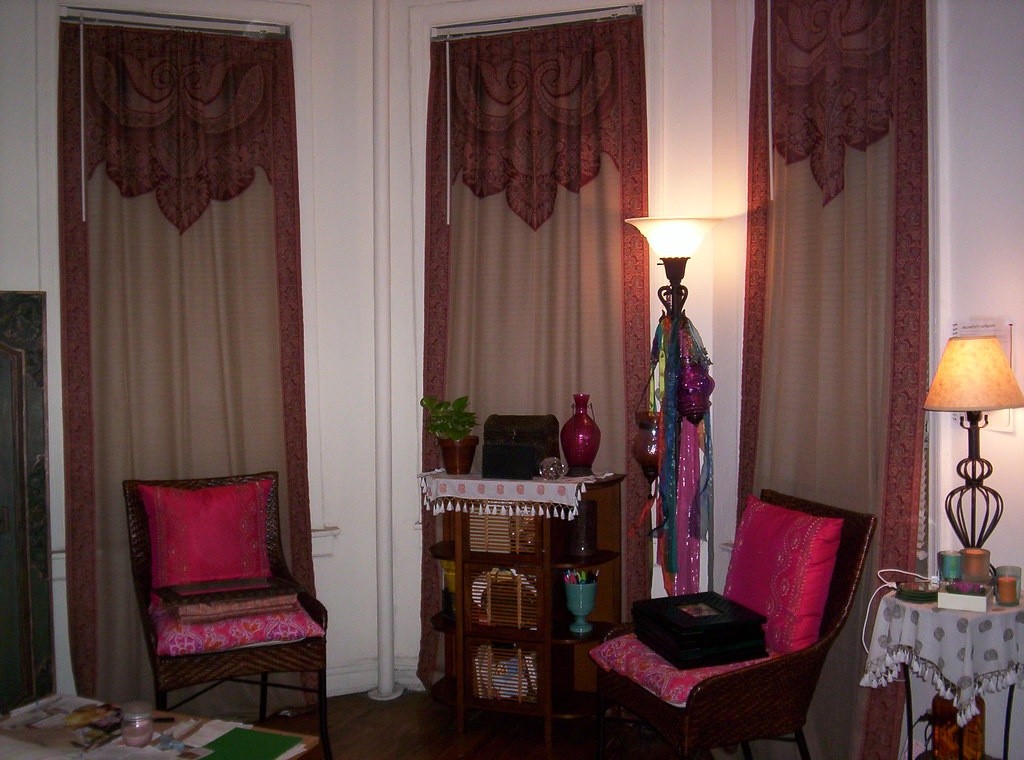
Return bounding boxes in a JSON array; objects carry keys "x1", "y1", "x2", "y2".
[
  {"x1": 937, "y1": 551, "x2": 962, "y2": 581},
  {"x1": 959, "y1": 548, "x2": 990, "y2": 583},
  {"x1": 996, "y1": 566, "x2": 1021, "y2": 606}
]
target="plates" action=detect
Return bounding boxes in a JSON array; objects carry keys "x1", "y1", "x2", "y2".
[{"x1": 895, "y1": 588, "x2": 937, "y2": 602}]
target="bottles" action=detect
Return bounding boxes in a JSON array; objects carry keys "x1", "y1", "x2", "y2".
[
  {"x1": 121, "y1": 711, "x2": 153, "y2": 747},
  {"x1": 560, "y1": 394, "x2": 601, "y2": 476}
]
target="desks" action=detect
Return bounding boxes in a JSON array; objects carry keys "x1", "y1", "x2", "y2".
[
  {"x1": 0, "y1": 694, "x2": 322, "y2": 760},
  {"x1": 883, "y1": 589, "x2": 1023, "y2": 760}
]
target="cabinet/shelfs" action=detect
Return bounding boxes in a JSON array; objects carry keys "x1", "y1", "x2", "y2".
[{"x1": 421, "y1": 468, "x2": 625, "y2": 742}]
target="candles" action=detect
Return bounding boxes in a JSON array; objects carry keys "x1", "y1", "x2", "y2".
[
  {"x1": 963, "y1": 549, "x2": 984, "y2": 578},
  {"x1": 943, "y1": 552, "x2": 961, "y2": 578},
  {"x1": 997, "y1": 576, "x2": 1017, "y2": 603}
]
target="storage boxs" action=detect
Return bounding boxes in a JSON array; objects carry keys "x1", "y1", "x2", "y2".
[
  {"x1": 631, "y1": 590, "x2": 769, "y2": 670},
  {"x1": 481, "y1": 414, "x2": 560, "y2": 480}
]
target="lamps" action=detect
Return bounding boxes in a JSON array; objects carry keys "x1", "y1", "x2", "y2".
[
  {"x1": 625, "y1": 216, "x2": 714, "y2": 324},
  {"x1": 923, "y1": 336, "x2": 1024, "y2": 586}
]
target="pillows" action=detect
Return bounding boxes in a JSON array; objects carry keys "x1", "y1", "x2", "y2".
[
  {"x1": 149, "y1": 594, "x2": 328, "y2": 657},
  {"x1": 719, "y1": 491, "x2": 845, "y2": 657},
  {"x1": 589, "y1": 630, "x2": 779, "y2": 707},
  {"x1": 136, "y1": 480, "x2": 274, "y2": 587}
]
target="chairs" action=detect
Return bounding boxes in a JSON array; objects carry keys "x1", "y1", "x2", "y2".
[
  {"x1": 593, "y1": 488, "x2": 878, "y2": 759},
  {"x1": 123, "y1": 471, "x2": 331, "y2": 760}
]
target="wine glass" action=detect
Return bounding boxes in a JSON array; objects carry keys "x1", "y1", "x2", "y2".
[{"x1": 566, "y1": 583, "x2": 596, "y2": 633}]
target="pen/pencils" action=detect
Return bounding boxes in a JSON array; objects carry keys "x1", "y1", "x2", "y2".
[{"x1": 562, "y1": 566, "x2": 600, "y2": 585}]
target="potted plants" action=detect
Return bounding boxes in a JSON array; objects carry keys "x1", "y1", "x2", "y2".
[{"x1": 418, "y1": 394, "x2": 479, "y2": 476}]
